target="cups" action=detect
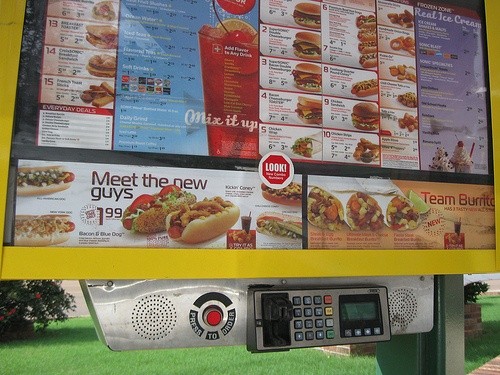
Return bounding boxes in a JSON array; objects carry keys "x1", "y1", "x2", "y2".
[
  {"x1": 241, "y1": 216, "x2": 251, "y2": 233},
  {"x1": 197, "y1": 31, "x2": 258, "y2": 160},
  {"x1": 454, "y1": 222, "x2": 460, "y2": 235},
  {"x1": 430, "y1": 163, "x2": 472, "y2": 173}
]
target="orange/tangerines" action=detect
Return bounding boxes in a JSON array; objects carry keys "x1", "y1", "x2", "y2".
[{"x1": 216, "y1": 19, "x2": 259, "y2": 74}]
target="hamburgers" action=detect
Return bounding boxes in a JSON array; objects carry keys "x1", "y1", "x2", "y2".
[
  {"x1": 291, "y1": 63, "x2": 322, "y2": 92},
  {"x1": 351, "y1": 102, "x2": 378, "y2": 131},
  {"x1": 292, "y1": 2, "x2": 321, "y2": 28},
  {"x1": 84, "y1": 0, "x2": 116, "y2": 76},
  {"x1": 292, "y1": 32, "x2": 321, "y2": 60}
]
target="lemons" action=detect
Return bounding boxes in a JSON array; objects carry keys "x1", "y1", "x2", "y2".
[{"x1": 407, "y1": 190, "x2": 431, "y2": 215}]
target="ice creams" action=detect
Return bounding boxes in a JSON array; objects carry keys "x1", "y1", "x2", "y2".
[
  {"x1": 429, "y1": 146, "x2": 450, "y2": 172},
  {"x1": 449, "y1": 140, "x2": 472, "y2": 172}
]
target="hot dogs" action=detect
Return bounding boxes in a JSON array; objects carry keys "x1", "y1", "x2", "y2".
[
  {"x1": 358, "y1": 41, "x2": 377, "y2": 54},
  {"x1": 350, "y1": 79, "x2": 378, "y2": 97},
  {"x1": 357, "y1": 28, "x2": 376, "y2": 41},
  {"x1": 256, "y1": 212, "x2": 301, "y2": 239},
  {"x1": 121, "y1": 184, "x2": 196, "y2": 234},
  {"x1": 356, "y1": 14, "x2": 376, "y2": 29},
  {"x1": 15, "y1": 215, "x2": 74, "y2": 246},
  {"x1": 16, "y1": 165, "x2": 75, "y2": 195},
  {"x1": 165, "y1": 196, "x2": 240, "y2": 243},
  {"x1": 358, "y1": 52, "x2": 377, "y2": 68},
  {"x1": 261, "y1": 182, "x2": 304, "y2": 206}
]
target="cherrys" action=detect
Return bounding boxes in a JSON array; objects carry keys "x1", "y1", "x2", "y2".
[{"x1": 213, "y1": 0, "x2": 250, "y2": 42}]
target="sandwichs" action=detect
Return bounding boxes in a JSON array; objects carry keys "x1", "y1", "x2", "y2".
[{"x1": 295, "y1": 96, "x2": 322, "y2": 124}]
